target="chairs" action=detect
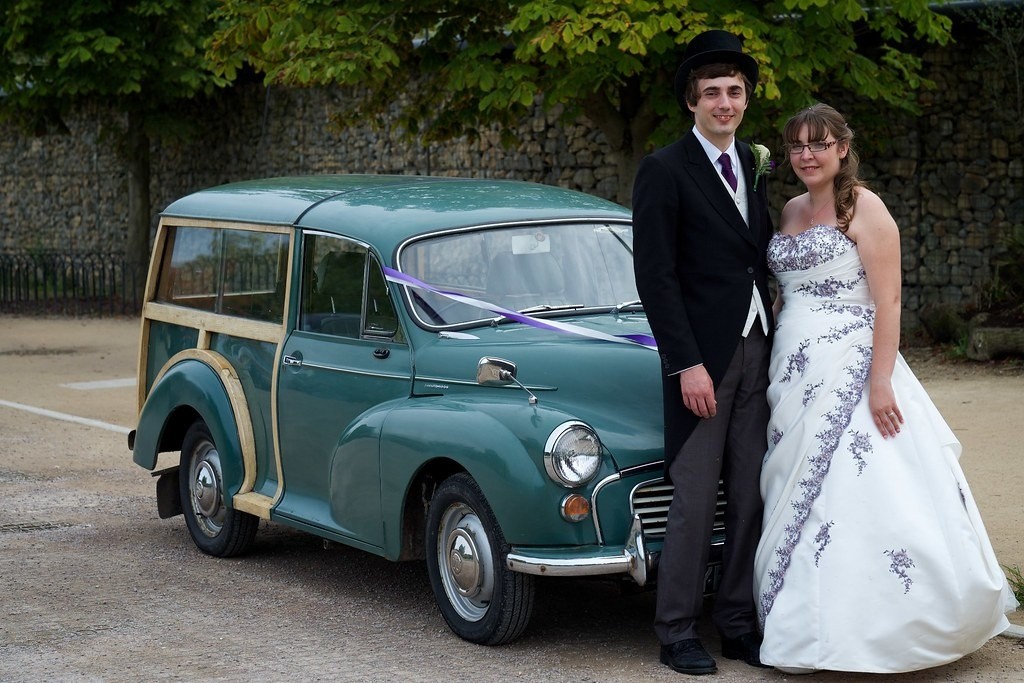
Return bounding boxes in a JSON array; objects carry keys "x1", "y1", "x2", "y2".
[
  {"x1": 310, "y1": 251, "x2": 395, "y2": 312},
  {"x1": 479, "y1": 248, "x2": 568, "y2": 323}
]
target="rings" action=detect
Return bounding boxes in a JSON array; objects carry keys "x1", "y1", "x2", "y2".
[{"x1": 888, "y1": 412, "x2": 893, "y2": 416}]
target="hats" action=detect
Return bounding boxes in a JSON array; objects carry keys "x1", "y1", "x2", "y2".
[{"x1": 676, "y1": 29, "x2": 759, "y2": 118}]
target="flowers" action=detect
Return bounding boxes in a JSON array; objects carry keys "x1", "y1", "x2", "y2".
[{"x1": 749, "y1": 141, "x2": 772, "y2": 192}]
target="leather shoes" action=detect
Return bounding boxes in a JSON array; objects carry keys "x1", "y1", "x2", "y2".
[
  {"x1": 659, "y1": 638, "x2": 718, "y2": 674},
  {"x1": 724, "y1": 630, "x2": 771, "y2": 668}
]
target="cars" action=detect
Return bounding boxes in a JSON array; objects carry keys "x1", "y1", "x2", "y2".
[{"x1": 127, "y1": 173, "x2": 726, "y2": 648}]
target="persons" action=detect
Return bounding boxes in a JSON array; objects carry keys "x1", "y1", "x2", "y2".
[
  {"x1": 631, "y1": 30, "x2": 776, "y2": 672},
  {"x1": 751, "y1": 103, "x2": 1021, "y2": 674}
]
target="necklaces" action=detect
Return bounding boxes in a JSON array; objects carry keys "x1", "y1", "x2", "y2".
[{"x1": 810, "y1": 195, "x2": 835, "y2": 224}]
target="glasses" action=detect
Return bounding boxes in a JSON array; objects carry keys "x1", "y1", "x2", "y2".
[{"x1": 787, "y1": 138, "x2": 844, "y2": 155}]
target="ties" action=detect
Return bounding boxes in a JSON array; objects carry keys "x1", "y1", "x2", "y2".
[{"x1": 717, "y1": 152, "x2": 738, "y2": 193}]
